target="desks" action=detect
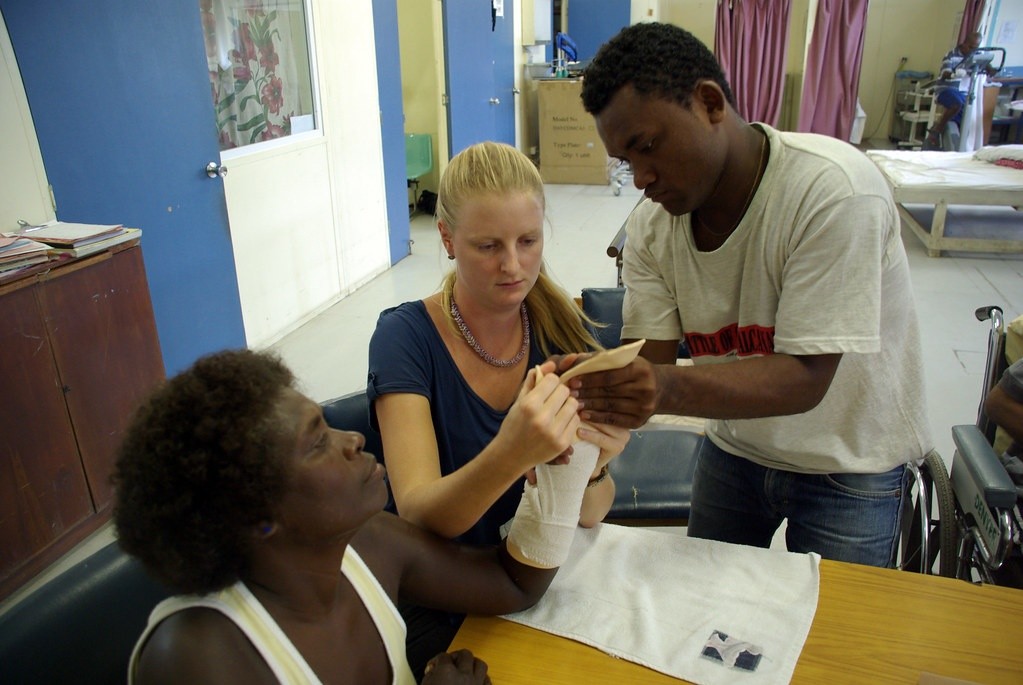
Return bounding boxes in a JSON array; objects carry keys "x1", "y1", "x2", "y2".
[{"x1": 445, "y1": 523, "x2": 1023, "y2": 684}]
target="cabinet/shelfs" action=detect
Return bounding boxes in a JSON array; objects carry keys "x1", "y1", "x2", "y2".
[{"x1": 0, "y1": 237, "x2": 166, "y2": 603}]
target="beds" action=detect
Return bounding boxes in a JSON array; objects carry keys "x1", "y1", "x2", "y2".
[{"x1": 866, "y1": 149, "x2": 1023, "y2": 256}]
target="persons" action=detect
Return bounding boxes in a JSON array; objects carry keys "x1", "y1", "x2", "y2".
[
  {"x1": 365, "y1": 140, "x2": 631, "y2": 676},
  {"x1": 986, "y1": 352, "x2": 1023, "y2": 592},
  {"x1": 561, "y1": 19, "x2": 931, "y2": 568},
  {"x1": 929, "y1": 31, "x2": 994, "y2": 148},
  {"x1": 111, "y1": 348, "x2": 626, "y2": 685}
]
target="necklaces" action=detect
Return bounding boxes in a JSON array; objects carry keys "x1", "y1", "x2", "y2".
[{"x1": 447, "y1": 284, "x2": 530, "y2": 368}]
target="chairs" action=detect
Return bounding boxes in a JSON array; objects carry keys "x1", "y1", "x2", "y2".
[
  {"x1": 318, "y1": 388, "x2": 396, "y2": 517},
  {"x1": 0, "y1": 540, "x2": 171, "y2": 685},
  {"x1": 406, "y1": 134, "x2": 434, "y2": 221},
  {"x1": 580, "y1": 287, "x2": 704, "y2": 529}
]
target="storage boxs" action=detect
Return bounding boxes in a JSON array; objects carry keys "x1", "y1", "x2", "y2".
[{"x1": 537, "y1": 80, "x2": 611, "y2": 186}]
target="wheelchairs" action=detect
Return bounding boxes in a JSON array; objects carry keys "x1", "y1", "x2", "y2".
[{"x1": 895, "y1": 306, "x2": 1023, "y2": 587}]
